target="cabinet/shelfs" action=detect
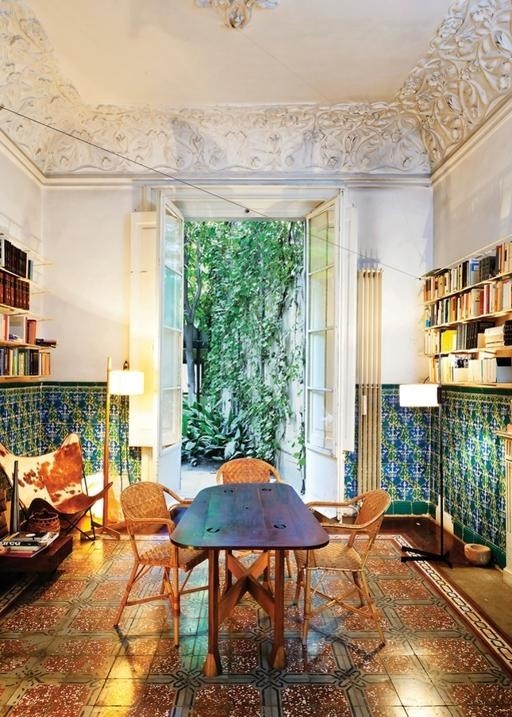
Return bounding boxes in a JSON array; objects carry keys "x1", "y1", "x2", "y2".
[
  {"x1": 420, "y1": 234, "x2": 512, "y2": 390},
  {"x1": 0, "y1": 227, "x2": 57, "y2": 382}
]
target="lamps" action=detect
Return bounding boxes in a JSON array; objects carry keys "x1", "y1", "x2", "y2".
[
  {"x1": 78, "y1": 356, "x2": 146, "y2": 541},
  {"x1": 397, "y1": 384, "x2": 455, "y2": 571}
]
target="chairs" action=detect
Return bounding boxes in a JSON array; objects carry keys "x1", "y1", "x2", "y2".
[
  {"x1": 215, "y1": 457, "x2": 294, "y2": 579},
  {"x1": 0, "y1": 429, "x2": 113, "y2": 542},
  {"x1": 112, "y1": 481, "x2": 211, "y2": 646},
  {"x1": 293, "y1": 488, "x2": 393, "y2": 649}
]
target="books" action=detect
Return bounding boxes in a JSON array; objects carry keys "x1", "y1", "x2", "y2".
[
  {"x1": 0, "y1": 235, "x2": 56, "y2": 377},
  {"x1": 424, "y1": 241, "x2": 512, "y2": 385},
  {"x1": 0, "y1": 530, "x2": 58, "y2": 558}
]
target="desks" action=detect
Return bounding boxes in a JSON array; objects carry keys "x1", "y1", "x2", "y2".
[{"x1": 168, "y1": 481, "x2": 329, "y2": 678}]
